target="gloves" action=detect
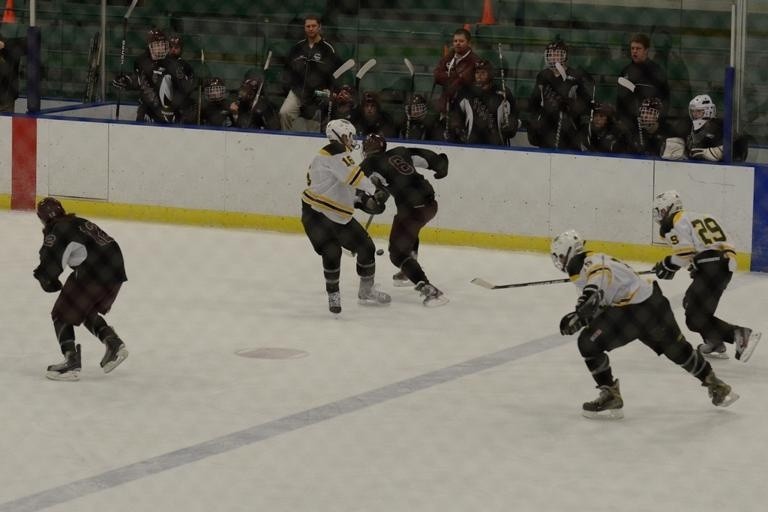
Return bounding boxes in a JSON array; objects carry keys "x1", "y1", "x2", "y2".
[
  {"x1": 439, "y1": 111, "x2": 458, "y2": 126},
  {"x1": 528, "y1": 116, "x2": 544, "y2": 131},
  {"x1": 573, "y1": 131, "x2": 594, "y2": 151},
  {"x1": 248, "y1": 100, "x2": 267, "y2": 117},
  {"x1": 542, "y1": 86, "x2": 562, "y2": 115},
  {"x1": 559, "y1": 311, "x2": 588, "y2": 335},
  {"x1": 653, "y1": 255, "x2": 681, "y2": 280},
  {"x1": 441, "y1": 127, "x2": 455, "y2": 141},
  {"x1": 113, "y1": 74, "x2": 133, "y2": 90},
  {"x1": 501, "y1": 115, "x2": 518, "y2": 138}
]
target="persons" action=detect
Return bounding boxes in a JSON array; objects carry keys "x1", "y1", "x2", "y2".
[
  {"x1": 350, "y1": 133, "x2": 451, "y2": 310},
  {"x1": 32, "y1": 197, "x2": 128, "y2": 375},
  {"x1": 649, "y1": 191, "x2": 762, "y2": 363},
  {"x1": 300, "y1": 117, "x2": 392, "y2": 313},
  {"x1": 111, "y1": 14, "x2": 728, "y2": 167},
  {"x1": 0, "y1": 36, "x2": 21, "y2": 113},
  {"x1": 547, "y1": 229, "x2": 738, "y2": 417}
]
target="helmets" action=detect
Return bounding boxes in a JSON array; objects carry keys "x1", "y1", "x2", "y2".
[
  {"x1": 326, "y1": 119, "x2": 359, "y2": 152},
  {"x1": 407, "y1": 94, "x2": 429, "y2": 120},
  {"x1": 335, "y1": 85, "x2": 381, "y2": 117},
  {"x1": 472, "y1": 60, "x2": 493, "y2": 87},
  {"x1": 36, "y1": 197, "x2": 64, "y2": 221},
  {"x1": 146, "y1": 28, "x2": 184, "y2": 59},
  {"x1": 544, "y1": 41, "x2": 569, "y2": 69},
  {"x1": 203, "y1": 76, "x2": 265, "y2": 102},
  {"x1": 587, "y1": 95, "x2": 717, "y2": 129}
]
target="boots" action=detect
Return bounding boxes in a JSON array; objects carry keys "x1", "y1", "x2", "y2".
[
  {"x1": 415, "y1": 281, "x2": 444, "y2": 304},
  {"x1": 358, "y1": 278, "x2": 392, "y2": 303},
  {"x1": 698, "y1": 338, "x2": 726, "y2": 353},
  {"x1": 393, "y1": 269, "x2": 411, "y2": 281},
  {"x1": 733, "y1": 325, "x2": 752, "y2": 361},
  {"x1": 582, "y1": 379, "x2": 624, "y2": 411},
  {"x1": 327, "y1": 289, "x2": 342, "y2": 313},
  {"x1": 701, "y1": 369, "x2": 732, "y2": 407},
  {"x1": 47, "y1": 344, "x2": 82, "y2": 372},
  {"x1": 100, "y1": 326, "x2": 125, "y2": 368}
]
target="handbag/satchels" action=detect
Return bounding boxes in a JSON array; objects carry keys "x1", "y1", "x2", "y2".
[{"x1": 183, "y1": 103, "x2": 199, "y2": 119}]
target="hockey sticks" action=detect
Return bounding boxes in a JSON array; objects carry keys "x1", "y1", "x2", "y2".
[
  {"x1": 345, "y1": 214, "x2": 376, "y2": 257},
  {"x1": 554, "y1": 60, "x2": 567, "y2": 152},
  {"x1": 349, "y1": 59, "x2": 377, "y2": 132},
  {"x1": 403, "y1": 57, "x2": 415, "y2": 140},
  {"x1": 617, "y1": 77, "x2": 644, "y2": 144},
  {"x1": 470, "y1": 268, "x2": 656, "y2": 290},
  {"x1": 324, "y1": 59, "x2": 355, "y2": 123},
  {"x1": 115, "y1": 1, "x2": 139, "y2": 120}
]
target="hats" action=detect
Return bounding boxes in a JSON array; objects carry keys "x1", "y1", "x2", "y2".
[
  {"x1": 653, "y1": 192, "x2": 683, "y2": 227},
  {"x1": 362, "y1": 133, "x2": 387, "y2": 158},
  {"x1": 551, "y1": 230, "x2": 585, "y2": 274}
]
